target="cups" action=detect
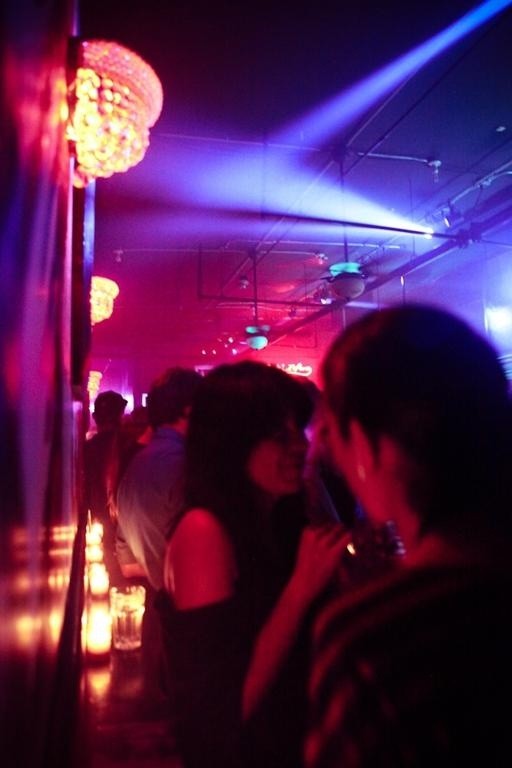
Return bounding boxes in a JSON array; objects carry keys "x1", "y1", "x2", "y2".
[{"x1": 109, "y1": 585, "x2": 146, "y2": 651}]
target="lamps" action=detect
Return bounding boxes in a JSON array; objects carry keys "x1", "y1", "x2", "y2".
[{"x1": 64, "y1": 39, "x2": 164, "y2": 189}]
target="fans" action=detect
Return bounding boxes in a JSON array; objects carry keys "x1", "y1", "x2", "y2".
[{"x1": 281, "y1": 148, "x2": 410, "y2": 308}]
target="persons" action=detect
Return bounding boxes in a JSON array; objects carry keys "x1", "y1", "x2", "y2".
[{"x1": 84, "y1": 302, "x2": 511, "y2": 767}]
